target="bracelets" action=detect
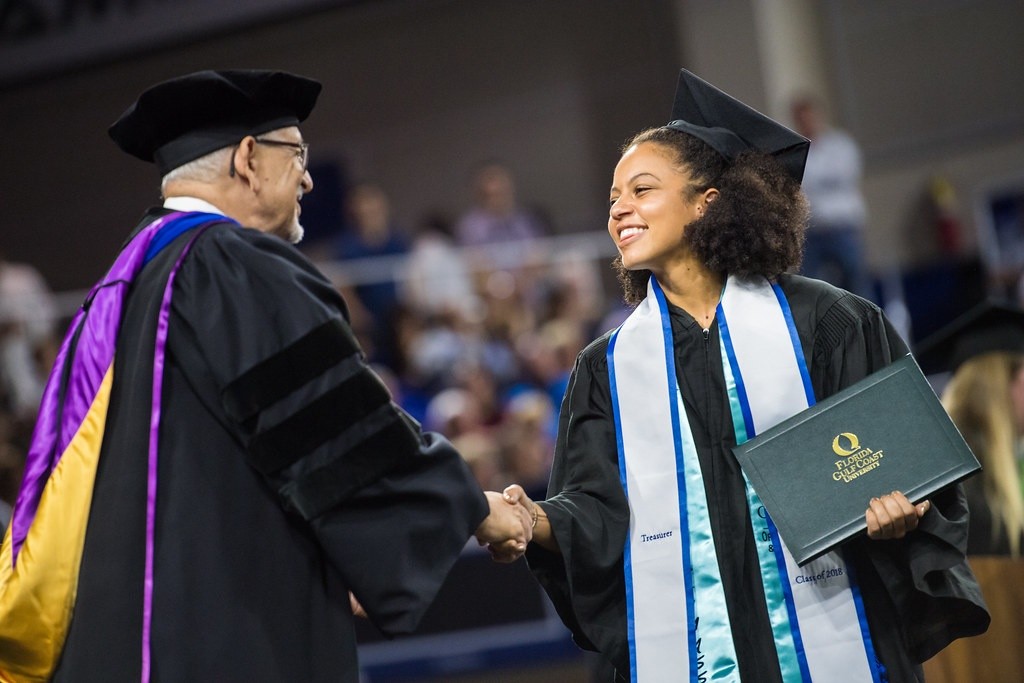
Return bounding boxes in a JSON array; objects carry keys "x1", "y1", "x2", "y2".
[{"x1": 532, "y1": 507, "x2": 537, "y2": 528}]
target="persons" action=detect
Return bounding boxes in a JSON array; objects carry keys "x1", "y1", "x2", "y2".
[
  {"x1": 503, "y1": 67, "x2": 991, "y2": 683},
  {"x1": 0, "y1": 70, "x2": 533, "y2": 683},
  {"x1": 898, "y1": 191, "x2": 1024, "y2": 556},
  {"x1": 785, "y1": 89, "x2": 878, "y2": 299},
  {"x1": 322, "y1": 162, "x2": 636, "y2": 492}
]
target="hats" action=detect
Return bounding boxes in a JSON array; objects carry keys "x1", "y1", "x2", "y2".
[
  {"x1": 661, "y1": 68, "x2": 811, "y2": 192},
  {"x1": 107, "y1": 71, "x2": 321, "y2": 179}
]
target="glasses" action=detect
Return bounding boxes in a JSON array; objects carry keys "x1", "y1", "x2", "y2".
[{"x1": 230, "y1": 138, "x2": 309, "y2": 177}]
